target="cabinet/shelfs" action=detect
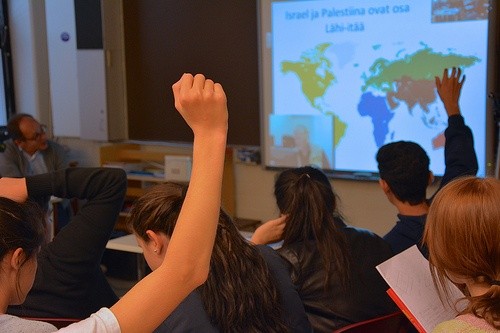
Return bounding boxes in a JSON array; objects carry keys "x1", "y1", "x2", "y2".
[{"x1": 99, "y1": 143, "x2": 236, "y2": 234}]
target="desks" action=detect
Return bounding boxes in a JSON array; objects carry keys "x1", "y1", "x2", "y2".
[{"x1": 106, "y1": 231, "x2": 284, "y2": 280}]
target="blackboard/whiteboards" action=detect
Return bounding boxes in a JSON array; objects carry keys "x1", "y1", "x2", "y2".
[{"x1": 118, "y1": 0, "x2": 260, "y2": 150}]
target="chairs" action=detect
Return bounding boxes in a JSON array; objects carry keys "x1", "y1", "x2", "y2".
[{"x1": 334, "y1": 311, "x2": 417, "y2": 333}]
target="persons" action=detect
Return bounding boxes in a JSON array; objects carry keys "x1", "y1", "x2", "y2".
[
  {"x1": 0, "y1": 72, "x2": 228, "y2": 333},
  {"x1": 129, "y1": 183, "x2": 312, "y2": 333},
  {"x1": 0, "y1": 113, "x2": 78, "y2": 178},
  {"x1": 249, "y1": 167, "x2": 396, "y2": 333},
  {"x1": 421, "y1": 175, "x2": 500, "y2": 333},
  {"x1": 376, "y1": 67, "x2": 478, "y2": 260},
  {"x1": 0, "y1": 166, "x2": 127, "y2": 319}
]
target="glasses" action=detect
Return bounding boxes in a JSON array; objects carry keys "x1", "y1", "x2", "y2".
[{"x1": 26, "y1": 125, "x2": 48, "y2": 141}]
